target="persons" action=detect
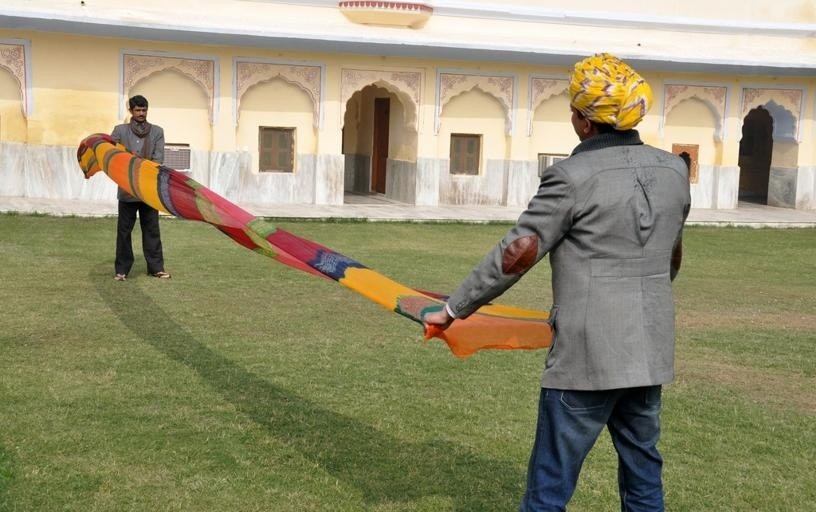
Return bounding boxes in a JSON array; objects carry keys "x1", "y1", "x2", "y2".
[
  {"x1": 108, "y1": 94, "x2": 173, "y2": 282},
  {"x1": 421, "y1": 52, "x2": 694, "y2": 512}
]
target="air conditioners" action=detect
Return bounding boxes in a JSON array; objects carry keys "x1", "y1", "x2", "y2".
[
  {"x1": 540, "y1": 156, "x2": 568, "y2": 177},
  {"x1": 162, "y1": 145, "x2": 192, "y2": 173}
]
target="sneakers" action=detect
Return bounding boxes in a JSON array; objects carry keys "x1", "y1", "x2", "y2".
[
  {"x1": 115, "y1": 273, "x2": 127, "y2": 282},
  {"x1": 147, "y1": 270, "x2": 172, "y2": 279}
]
